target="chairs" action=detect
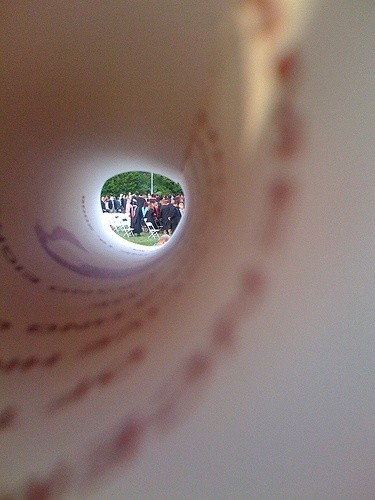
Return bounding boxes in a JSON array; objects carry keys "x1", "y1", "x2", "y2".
[{"x1": 103, "y1": 212, "x2": 164, "y2": 240}]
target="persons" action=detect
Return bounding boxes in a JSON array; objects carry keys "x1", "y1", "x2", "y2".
[{"x1": 100, "y1": 191, "x2": 185, "y2": 247}]
